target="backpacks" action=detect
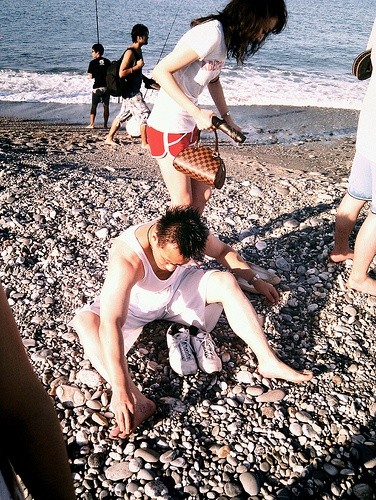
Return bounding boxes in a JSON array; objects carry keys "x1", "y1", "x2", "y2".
[
  {"x1": 93, "y1": 59, "x2": 108, "y2": 89},
  {"x1": 106, "y1": 48, "x2": 140, "y2": 97}
]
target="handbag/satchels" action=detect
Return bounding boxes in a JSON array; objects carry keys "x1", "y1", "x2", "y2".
[{"x1": 172, "y1": 127, "x2": 226, "y2": 189}]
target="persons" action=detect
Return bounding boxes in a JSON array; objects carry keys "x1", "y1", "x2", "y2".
[
  {"x1": 0, "y1": 284, "x2": 77, "y2": 500},
  {"x1": 71, "y1": 206, "x2": 313, "y2": 436},
  {"x1": 86, "y1": 43, "x2": 112, "y2": 128},
  {"x1": 105, "y1": 24, "x2": 156, "y2": 149},
  {"x1": 330, "y1": 14, "x2": 376, "y2": 296},
  {"x1": 147, "y1": 0, "x2": 288, "y2": 219}
]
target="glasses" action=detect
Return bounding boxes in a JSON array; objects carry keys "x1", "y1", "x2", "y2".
[{"x1": 90, "y1": 50, "x2": 99, "y2": 52}]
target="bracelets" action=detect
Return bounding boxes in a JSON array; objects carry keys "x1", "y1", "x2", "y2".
[
  {"x1": 130, "y1": 68, "x2": 133, "y2": 73},
  {"x1": 222, "y1": 109, "x2": 231, "y2": 119},
  {"x1": 248, "y1": 273, "x2": 260, "y2": 285}
]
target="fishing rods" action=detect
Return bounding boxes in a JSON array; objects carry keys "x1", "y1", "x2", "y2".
[
  {"x1": 142, "y1": 0, "x2": 183, "y2": 101},
  {"x1": 95, "y1": 0, "x2": 99, "y2": 44}
]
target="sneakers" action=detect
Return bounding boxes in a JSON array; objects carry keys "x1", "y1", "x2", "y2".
[
  {"x1": 166, "y1": 322, "x2": 198, "y2": 376},
  {"x1": 189, "y1": 325, "x2": 223, "y2": 375}
]
